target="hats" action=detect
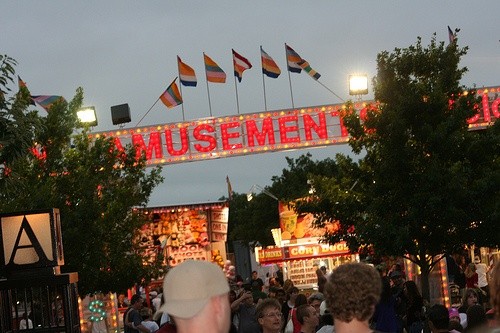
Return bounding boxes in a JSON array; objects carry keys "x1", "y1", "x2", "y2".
[
  {"x1": 153, "y1": 260, "x2": 230, "y2": 319},
  {"x1": 141, "y1": 321, "x2": 159, "y2": 332},
  {"x1": 474, "y1": 256, "x2": 480, "y2": 263},
  {"x1": 448, "y1": 308, "x2": 461, "y2": 323}
]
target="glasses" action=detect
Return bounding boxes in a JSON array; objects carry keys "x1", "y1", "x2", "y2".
[{"x1": 258, "y1": 312, "x2": 283, "y2": 319}]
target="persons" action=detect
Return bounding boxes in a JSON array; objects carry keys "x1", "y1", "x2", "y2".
[
  {"x1": 229, "y1": 266, "x2": 333, "y2": 333},
  {"x1": 161, "y1": 261, "x2": 231, "y2": 333},
  {"x1": 13, "y1": 296, "x2": 64, "y2": 329},
  {"x1": 118, "y1": 286, "x2": 171, "y2": 333},
  {"x1": 91, "y1": 313, "x2": 106, "y2": 333},
  {"x1": 324, "y1": 263, "x2": 382, "y2": 333},
  {"x1": 368, "y1": 256, "x2": 500, "y2": 333}
]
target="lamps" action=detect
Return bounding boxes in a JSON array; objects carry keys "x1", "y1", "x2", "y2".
[
  {"x1": 75, "y1": 106, "x2": 97, "y2": 133},
  {"x1": 111, "y1": 103, "x2": 131, "y2": 125},
  {"x1": 348, "y1": 74, "x2": 369, "y2": 100}
]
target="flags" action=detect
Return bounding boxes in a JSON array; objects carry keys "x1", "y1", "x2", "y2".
[
  {"x1": 18, "y1": 76, "x2": 63, "y2": 114},
  {"x1": 286, "y1": 45, "x2": 321, "y2": 80},
  {"x1": 160, "y1": 83, "x2": 183, "y2": 109},
  {"x1": 204, "y1": 55, "x2": 226, "y2": 83},
  {"x1": 261, "y1": 48, "x2": 281, "y2": 79},
  {"x1": 233, "y1": 50, "x2": 252, "y2": 83},
  {"x1": 178, "y1": 56, "x2": 197, "y2": 87}
]
